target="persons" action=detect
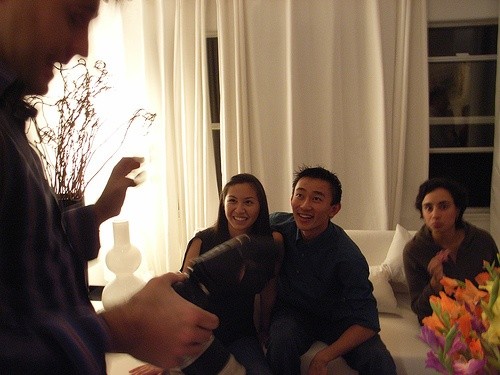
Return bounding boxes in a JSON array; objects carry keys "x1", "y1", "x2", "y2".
[
  {"x1": 129, "y1": 174, "x2": 285, "y2": 375},
  {"x1": 267, "y1": 167, "x2": 397, "y2": 375},
  {"x1": 403, "y1": 178, "x2": 500, "y2": 327},
  {"x1": 0, "y1": 0, "x2": 218, "y2": 375}
]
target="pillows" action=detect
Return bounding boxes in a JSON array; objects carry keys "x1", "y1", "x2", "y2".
[{"x1": 368, "y1": 223, "x2": 414, "y2": 318}]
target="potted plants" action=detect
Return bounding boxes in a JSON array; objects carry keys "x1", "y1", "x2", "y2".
[{"x1": 23, "y1": 58, "x2": 157, "y2": 214}]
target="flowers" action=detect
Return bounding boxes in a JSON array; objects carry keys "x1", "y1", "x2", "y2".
[{"x1": 416, "y1": 252, "x2": 500, "y2": 375}]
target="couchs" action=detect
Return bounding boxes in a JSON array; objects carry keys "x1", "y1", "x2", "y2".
[{"x1": 253, "y1": 229, "x2": 446, "y2": 375}]
